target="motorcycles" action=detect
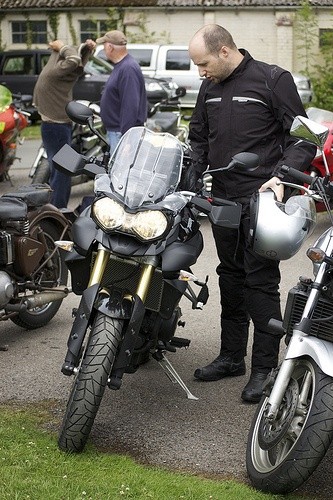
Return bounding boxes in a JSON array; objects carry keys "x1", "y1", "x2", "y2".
[
  {"x1": 0, "y1": 88, "x2": 260, "y2": 456},
  {"x1": 244, "y1": 114, "x2": 333, "y2": 495}
]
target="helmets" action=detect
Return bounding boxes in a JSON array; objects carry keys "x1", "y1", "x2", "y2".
[{"x1": 246, "y1": 188, "x2": 317, "y2": 260}]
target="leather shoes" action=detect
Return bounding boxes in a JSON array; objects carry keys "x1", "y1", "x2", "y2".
[
  {"x1": 241, "y1": 372, "x2": 267, "y2": 402},
  {"x1": 194, "y1": 355, "x2": 246, "y2": 381}
]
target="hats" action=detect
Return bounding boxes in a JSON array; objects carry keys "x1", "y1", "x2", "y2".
[{"x1": 96, "y1": 30, "x2": 128, "y2": 45}]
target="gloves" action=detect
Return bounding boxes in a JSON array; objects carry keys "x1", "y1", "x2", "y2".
[{"x1": 182, "y1": 163, "x2": 205, "y2": 192}]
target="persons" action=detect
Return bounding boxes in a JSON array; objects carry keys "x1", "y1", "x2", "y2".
[
  {"x1": 32, "y1": 40, "x2": 95, "y2": 209},
  {"x1": 95, "y1": 31, "x2": 148, "y2": 157},
  {"x1": 182, "y1": 24, "x2": 317, "y2": 402}
]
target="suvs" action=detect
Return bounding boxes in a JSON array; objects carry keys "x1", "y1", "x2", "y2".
[
  {"x1": 84, "y1": 43, "x2": 312, "y2": 112},
  {"x1": 0, "y1": 49, "x2": 170, "y2": 120}
]
